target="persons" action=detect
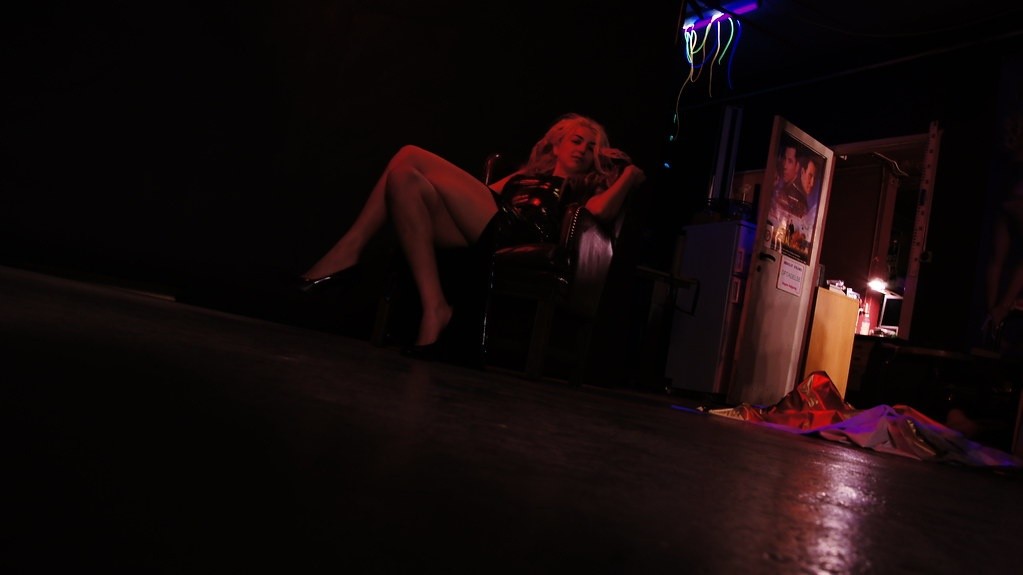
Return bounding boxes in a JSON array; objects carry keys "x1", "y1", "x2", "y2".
[
  {"x1": 763, "y1": 145, "x2": 815, "y2": 254},
  {"x1": 979, "y1": 61, "x2": 1023, "y2": 350},
  {"x1": 275, "y1": 112, "x2": 646, "y2": 359}
]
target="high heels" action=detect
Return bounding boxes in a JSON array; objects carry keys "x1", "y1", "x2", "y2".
[
  {"x1": 293, "y1": 263, "x2": 361, "y2": 291},
  {"x1": 401, "y1": 310, "x2": 459, "y2": 359}
]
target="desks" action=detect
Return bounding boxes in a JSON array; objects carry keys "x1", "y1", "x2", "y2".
[{"x1": 799, "y1": 286, "x2": 860, "y2": 399}]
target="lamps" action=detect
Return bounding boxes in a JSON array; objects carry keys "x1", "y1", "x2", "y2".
[{"x1": 866, "y1": 277, "x2": 904, "y2": 337}]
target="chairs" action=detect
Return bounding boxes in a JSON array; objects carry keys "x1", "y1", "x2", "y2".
[{"x1": 476, "y1": 201, "x2": 626, "y2": 380}]
